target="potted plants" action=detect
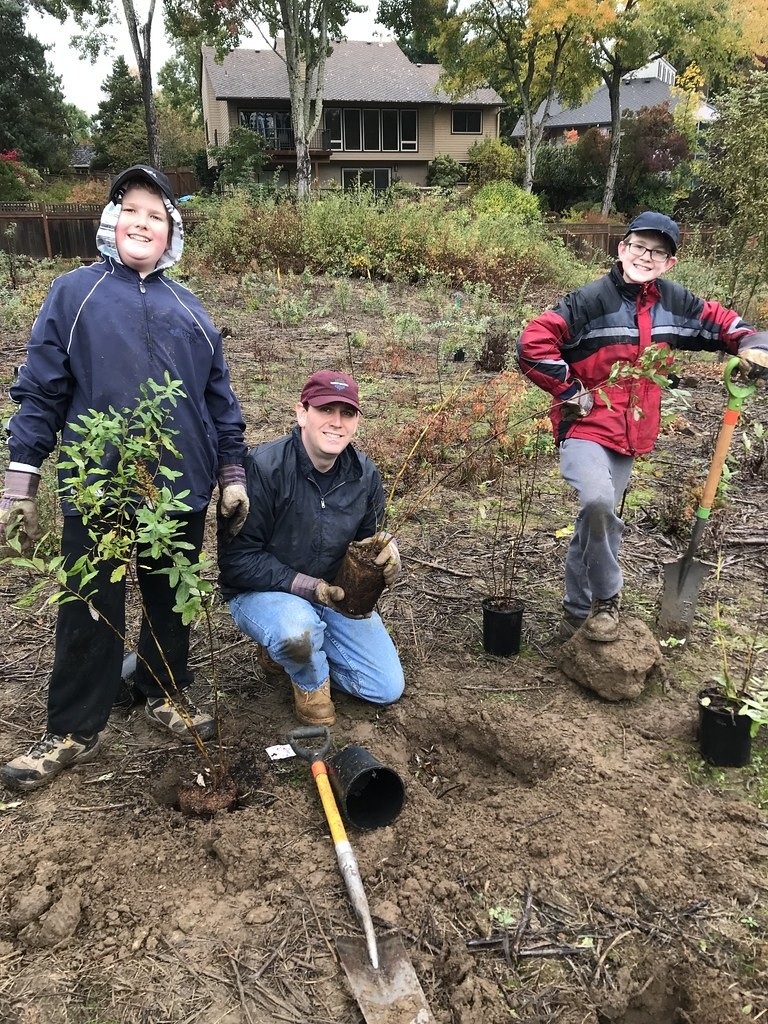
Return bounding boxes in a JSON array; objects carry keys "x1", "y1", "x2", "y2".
[{"x1": 698, "y1": 673, "x2": 768, "y2": 768}]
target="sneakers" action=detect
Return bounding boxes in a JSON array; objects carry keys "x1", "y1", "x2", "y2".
[
  {"x1": 142, "y1": 686, "x2": 219, "y2": 744},
  {"x1": 0, "y1": 729, "x2": 102, "y2": 792},
  {"x1": 556, "y1": 604, "x2": 590, "y2": 642},
  {"x1": 255, "y1": 639, "x2": 284, "y2": 677},
  {"x1": 287, "y1": 672, "x2": 337, "y2": 727},
  {"x1": 580, "y1": 590, "x2": 623, "y2": 642}
]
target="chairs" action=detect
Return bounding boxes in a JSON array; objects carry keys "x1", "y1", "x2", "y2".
[{"x1": 278, "y1": 133, "x2": 291, "y2": 150}]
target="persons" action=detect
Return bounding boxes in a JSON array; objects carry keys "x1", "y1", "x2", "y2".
[
  {"x1": 216, "y1": 370, "x2": 404, "y2": 727},
  {"x1": 516, "y1": 212, "x2": 768, "y2": 642},
  {"x1": 0, "y1": 165, "x2": 250, "y2": 793}
]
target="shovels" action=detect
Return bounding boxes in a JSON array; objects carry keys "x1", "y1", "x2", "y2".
[
  {"x1": 289, "y1": 725, "x2": 436, "y2": 1024},
  {"x1": 659, "y1": 359, "x2": 756, "y2": 658}
]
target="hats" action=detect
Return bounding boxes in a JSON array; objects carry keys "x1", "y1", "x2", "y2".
[
  {"x1": 620, "y1": 210, "x2": 682, "y2": 256},
  {"x1": 299, "y1": 369, "x2": 364, "y2": 416},
  {"x1": 107, "y1": 164, "x2": 176, "y2": 213}
]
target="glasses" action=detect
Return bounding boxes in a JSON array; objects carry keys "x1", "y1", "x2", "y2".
[{"x1": 623, "y1": 239, "x2": 672, "y2": 264}]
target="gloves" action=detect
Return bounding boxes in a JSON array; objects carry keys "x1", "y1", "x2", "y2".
[
  {"x1": 556, "y1": 377, "x2": 595, "y2": 418},
  {"x1": 0, "y1": 470, "x2": 45, "y2": 558},
  {"x1": 730, "y1": 347, "x2": 768, "y2": 388},
  {"x1": 216, "y1": 463, "x2": 251, "y2": 545},
  {"x1": 288, "y1": 570, "x2": 378, "y2": 620},
  {"x1": 348, "y1": 530, "x2": 402, "y2": 589}
]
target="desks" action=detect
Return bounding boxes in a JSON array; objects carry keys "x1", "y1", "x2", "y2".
[{"x1": 267, "y1": 138, "x2": 280, "y2": 149}]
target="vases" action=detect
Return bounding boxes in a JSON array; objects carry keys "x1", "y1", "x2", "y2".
[
  {"x1": 326, "y1": 745, "x2": 405, "y2": 831},
  {"x1": 481, "y1": 597, "x2": 525, "y2": 653}
]
